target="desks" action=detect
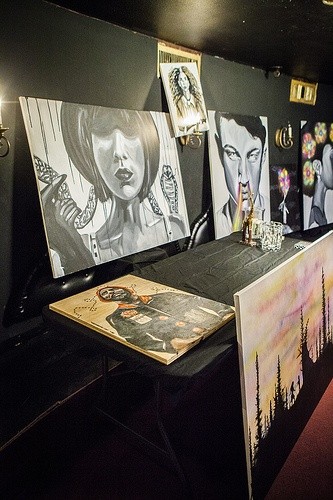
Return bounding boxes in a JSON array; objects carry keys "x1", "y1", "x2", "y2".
[{"x1": 41, "y1": 230, "x2": 312, "y2": 492}]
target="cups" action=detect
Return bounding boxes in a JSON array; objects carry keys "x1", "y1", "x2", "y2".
[
  {"x1": 260, "y1": 221, "x2": 282, "y2": 252},
  {"x1": 244, "y1": 206, "x2": 265, "y2": 239}
]
